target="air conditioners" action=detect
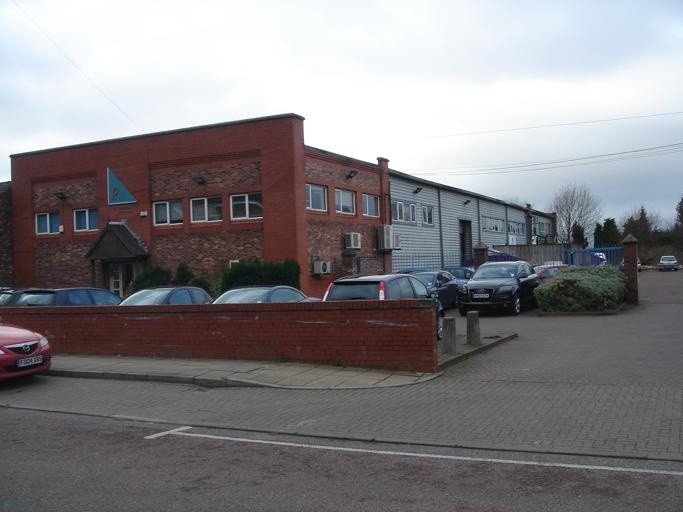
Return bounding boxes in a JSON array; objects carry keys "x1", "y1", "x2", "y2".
[
  {"x1": 313, "y1": 260, "x2": 331, "y2": 273},
  {"x1": 393, "y1": 234, "x2": 401, "y2": 249},
  {"x1": 376, "y1": 224, "x2": 393, "y2": 250},
  {"x1": 344, "y1": 232, "x2": 362, "y2": 249}
]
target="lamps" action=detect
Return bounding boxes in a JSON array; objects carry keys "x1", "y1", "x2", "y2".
[
  {"x1": 54, "y1": 192, "x2": 65, "y2": 200},
  {"x1": 464, "y1": 200, "x2": 470, "y2": 205},
  {"x1": 347, "y1": 171, "x2": 357, "y2": 179},
  {"x1": 413, "y1": 188, "x2": 422, "y2": 193},
  {"x1": 193, "y1": 176, "x2": 205, "y2": 184}
]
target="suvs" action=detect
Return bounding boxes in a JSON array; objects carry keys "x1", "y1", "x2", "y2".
[
  {"x1": 456, "y1": 261, "x2": 539, "y2": 315},
  {"x1": 322, "y1": 274, "x2": 445, "y2": 340}
]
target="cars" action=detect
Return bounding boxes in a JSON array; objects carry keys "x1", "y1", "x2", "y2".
[
  {"x1": 212, "y1": 285, "x2": 322, "y2": 304},
  {"x1": 396, "y1": 267, "x2": 476, "y2": 308},
  {"x1": 533, "y1": 264, "x2": 576, "y2": 284},
  {"x1": 620, "y1": 257, "x2": 641, "y2": 272},
  {"x1": 0, "y1": 286, "x2": 123, "y2": 306},
  {"x1": 0, "y1": 322, "x2": 51, "y2": 380},
  {"x1": 658, "y1": 256, "x2": 679, "y2": 271},
  {"x1": 119, "y1": 287, "x2": 214, "y2": 306}
]
